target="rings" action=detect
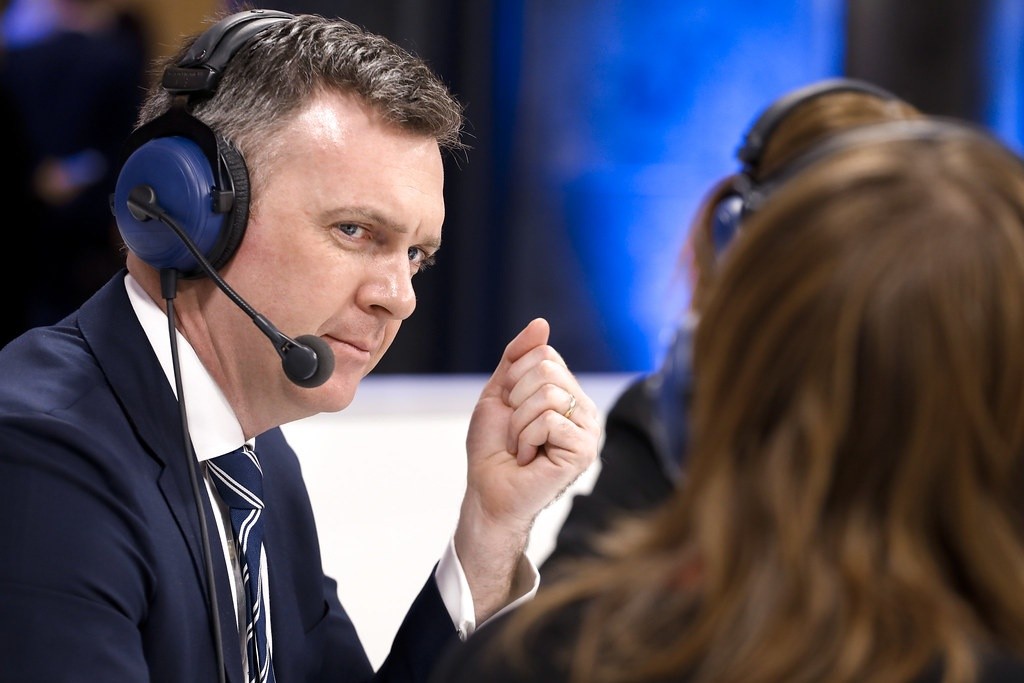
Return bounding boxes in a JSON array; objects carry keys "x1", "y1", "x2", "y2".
[{"x1": 564, "y1": 393, "x2": 577, "y2": 419}]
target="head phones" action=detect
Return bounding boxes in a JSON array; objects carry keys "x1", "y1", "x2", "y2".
[
  {"x1": 115, "y1": 8, "x2": 292, "y2": 278},
  {"x1": 653, "y1": 77, "x2": 896, "y2": 492}
]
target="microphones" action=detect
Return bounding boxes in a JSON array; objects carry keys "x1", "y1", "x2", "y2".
[{"x1": 149, "y1": 208, "x2": 335, "y2": 389}]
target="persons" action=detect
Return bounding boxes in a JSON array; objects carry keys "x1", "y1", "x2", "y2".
[
  {"x1": 430, "y1": 77, "x2": 1024, "y2": 683},
  {"x1": 0, "y1": 10, "x2": 600, "y2": 683},
  {"x1": 0, "y1": 0, "x2": 151, "y2": 355}
]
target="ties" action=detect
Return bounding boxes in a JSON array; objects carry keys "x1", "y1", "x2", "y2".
[{"x1": 206, "y1": 445, "x2": 277, "y2": 683}]
